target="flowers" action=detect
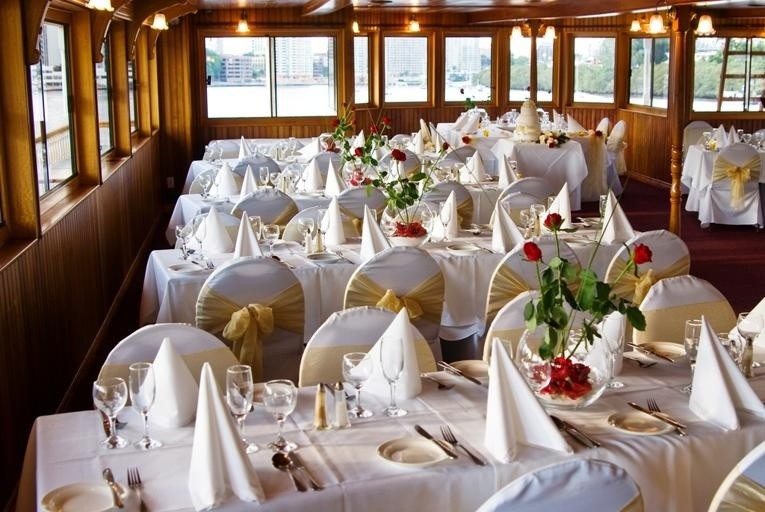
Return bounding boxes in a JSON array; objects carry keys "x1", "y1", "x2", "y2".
[{"x1": 330, "y1": 103, "x2": 355, "y2": 142}]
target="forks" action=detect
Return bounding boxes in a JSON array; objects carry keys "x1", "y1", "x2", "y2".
[
  {"x1": 438, "y1": 424, "x2": 485, "y2": 468},
  {"x1": 125, "y1": 466, "x2": 148, "y2": 512}
]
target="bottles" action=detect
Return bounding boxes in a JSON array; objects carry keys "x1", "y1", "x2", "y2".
[{"x1": 314, "y1": 380, "x2": 352, "y2": 430}]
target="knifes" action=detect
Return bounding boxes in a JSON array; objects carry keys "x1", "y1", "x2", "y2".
[
  {"x1": 288, "y1": 450, "x2": 324, "y2": 491},
  {"x1": 626, "y1": 341, "x2": 675, "y2": 364},
  {"x1": 437, "y1": 358, "x2": 481, "y2": 389},
  {"x1": 414, "y1": 423, "x2": 457, "y2": 460},
  {"x1": 626, "y1": 401, "x2": 687, "y2": 430}
]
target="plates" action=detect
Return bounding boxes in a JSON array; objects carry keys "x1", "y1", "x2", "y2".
[
  {"x1": 635, "y1": 342, "x2": 686, "y2": 359},
  {"x1": 40, "y1": 481, "x2": 129, "y2": 512},
  {"x1": 376, "y1": 436, "x2": 455, "y2": 468},
  {"x1": 450, "y1": 359, "x2": 490, "y2": 379},
  {"x1": 251, "y1": 382, "x2": 266, "y2": 405},
  {"x1": 607, "y1": 411, "x2": 679, "y2": 435}
]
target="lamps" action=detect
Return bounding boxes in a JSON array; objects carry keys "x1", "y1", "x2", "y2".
[
  {"x1": 352, "y1": 14, "x2": 360, "y2": 34},
  {"x1": 235, "y1": 8, "x2": 251, "y2": 33},
  {"x1": 150, "y1": 12, "x2": 169, "y2": 32},
  {"x1": 509, "y1": 17, "x2": 557, "y2": 44},
  {"x1": 628, "y1": 0, "x2": 717, "y2": 38},
  {"x1": 403, "y1": 14, "x2": 421, "y2": 33}
]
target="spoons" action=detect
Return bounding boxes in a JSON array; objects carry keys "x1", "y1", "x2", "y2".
[
  {"x1": 99, "y1": 468, "x2": 124, "y2": 510},
  {"x1": 422, "y1": 374, "x2": 456, "y2": 391},
  {"x1": 272, "y1": 453, "x2": 308, "y2": 492},
  {"x1": 549, "y1": 415, "x2": 594, "y2": 449}
]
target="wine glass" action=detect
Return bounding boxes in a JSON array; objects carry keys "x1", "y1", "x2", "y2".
[
  {"x1": 222, "y1": 364, "x2": 258, "y2": 455},
  {"x1": 518, "y1": 357, "x2": 551, "y2": 408},
  {"x1": 262, "y1": 379, "x2": 298, "y2": 452},
  {"x1": 701, "y1": 125, "x2": 765, "y2": 151},
  {"x1": 175, "y1": 137, "x2": 608, "y2": 252},
  {"x1": 681, "y1": 319, "x2": 705, "y2": 394},
  {"x1": 90, "y1": 361, "x2": 162, "y2": 452},
  {"x1": 342, "y1": 336, "x2": 408, "y2": 419},
  {"x1": 715, "y1": 313, "x2": 765, "y2": 380}
]
